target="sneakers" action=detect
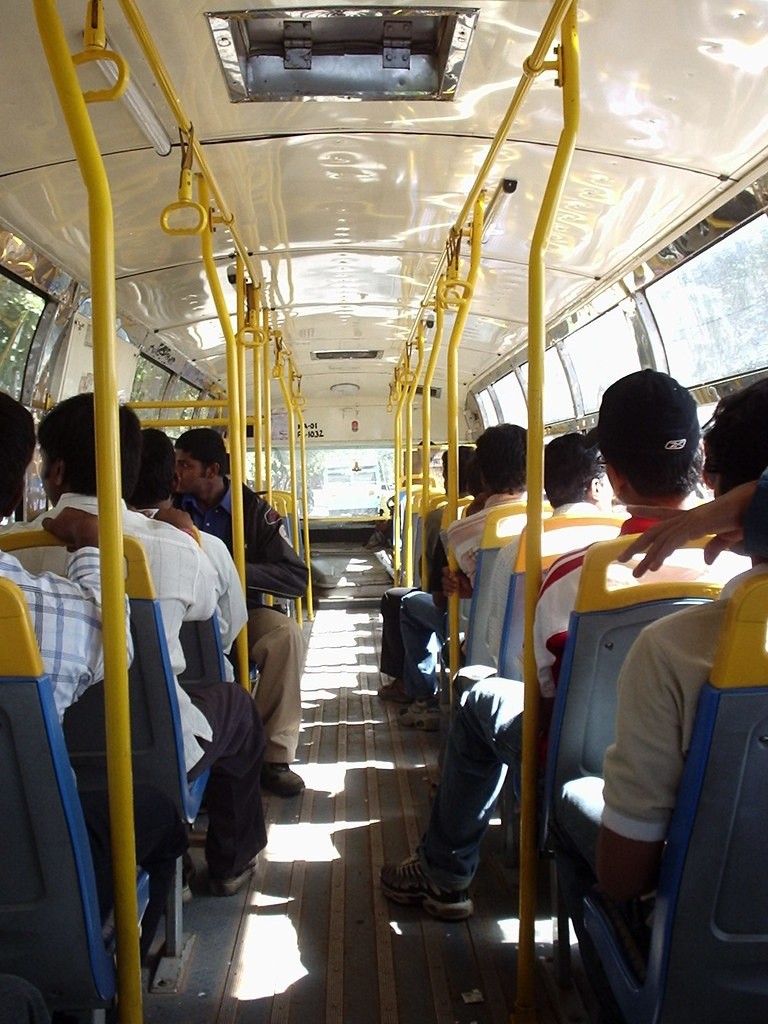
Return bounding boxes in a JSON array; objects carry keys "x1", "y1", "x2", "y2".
[
  {"x1": 400, "y1": 699, "x2": 440, "y2": 730},
  {"x1": 182, "y1": 851, "x2": 194, "y2": 903},
  {"x1": 379, "y1": 854, "x2": 474, "y2": 919},
  {"x1": 210, "y1": 854, "x2": 259, "y2": 895},
  {"x1": 378, "y1": 678, "x2": 429, "y2": 701},
  {"x1": 261, "y1": 760, "x2": 306, "y2": 794}
]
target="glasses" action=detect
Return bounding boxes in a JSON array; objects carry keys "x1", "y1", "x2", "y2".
[{"x1": 597, "y1": 455, "x2": 607, "y2": 468}]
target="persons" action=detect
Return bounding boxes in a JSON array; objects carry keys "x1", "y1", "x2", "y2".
[
  {"x1": 0, "y1": 392, "x2": 309, "y2": 1024},
  {"x1": 378, "y1": 368, "x2": 768, "y2": 980}
]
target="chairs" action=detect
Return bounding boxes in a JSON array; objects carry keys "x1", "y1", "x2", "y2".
[
  {"x1": 400, "y1": 484, "x2": 768, "y2": 1024},
  {"x1": 0, "y1": 490, "x2": 303, "y2": 1024}
]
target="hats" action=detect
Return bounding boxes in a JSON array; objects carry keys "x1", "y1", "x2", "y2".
[{"x1": 577, "y1": 369, "x2": 700, "y2": 452}]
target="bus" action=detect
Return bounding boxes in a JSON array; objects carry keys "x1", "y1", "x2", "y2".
[{"x1": 322, "y1": 463, "x2": 381, "y2": 517}]
